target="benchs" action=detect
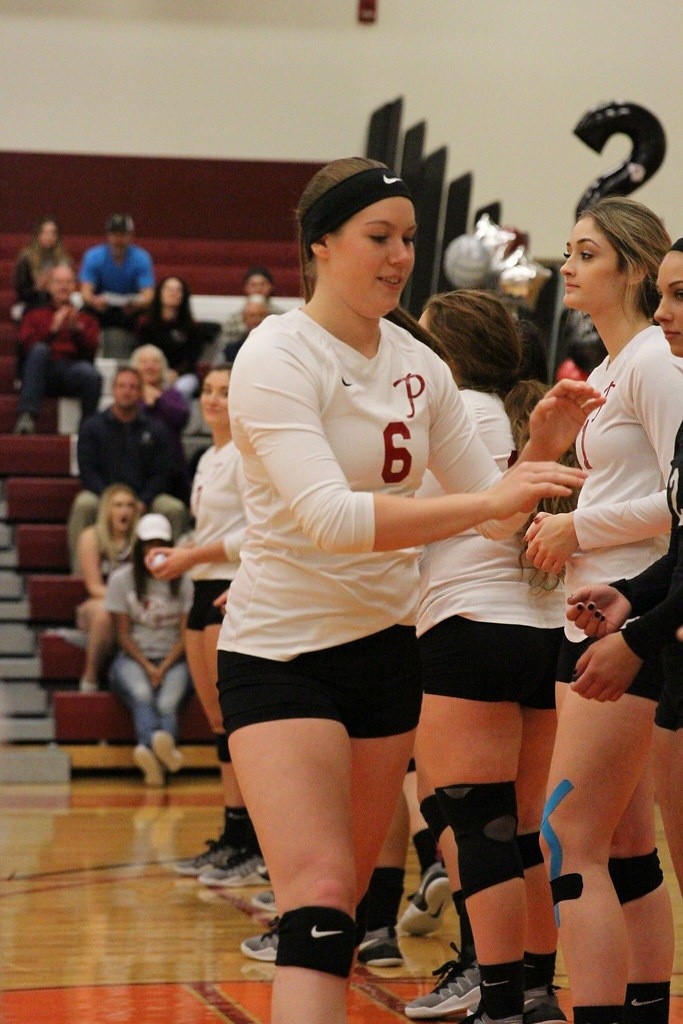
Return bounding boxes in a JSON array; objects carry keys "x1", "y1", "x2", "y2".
[{"x1": 0, "y1": 233, "x2": 306, "y2": 767}]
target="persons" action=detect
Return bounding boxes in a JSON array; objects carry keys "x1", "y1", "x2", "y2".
[
  {"x1": 134, "y1": 274, "x2": 200, "y2": 400},
  {"x1": 144, "y1": 361, "x2": 270, "y2": 888},
  {"x1": 10, "y1": 211, "x2": 156, "y2": 392},
  {"x1": 74, "y1": 483, "x2": 195, "y2": 785},
  {"x1": 63, "y1": 341, "x2": 190, "y2": 577},
  {"x1": 211, "y1": 294, "x2": 270, "y2": 367},
  {"x1": 200, "y1": 263, "x2": 285, "y2": 375},
  {"x1": 15, "y1": 262, "x2": 104, "y2": 434},
  {"x1": 215, "y1": 157, "x2": 683, "y2": 1024}
]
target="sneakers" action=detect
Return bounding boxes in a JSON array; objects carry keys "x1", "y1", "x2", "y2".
[
  {"x1": 250, "y1": 890, "x2": 274, "y2": 913},
  {"x1": 357, "y1": 932, "x2": 403, "y2": 966},
  {"x1": 174, "y1": 840, "x2": 248, "y2": 875},
  {"x1": 238, "y1": 923, "x2": 281, "y2": 962},
  {"x1": 198, "y1": 849, "x2": 270, "y2": 885},
  {"x1": 521, "y1": 984, "x2": 567, "y2": 1024},
  {"x1": 400, "y1": 868, "x2": 454, "y2": 937},
  {"x1": 405, "y1": 942, "x2": 483, "y2": 1016}
]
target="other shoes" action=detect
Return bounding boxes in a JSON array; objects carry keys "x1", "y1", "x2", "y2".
[
  {"x1": 133, "y1": 802, "x2": 159, "y2": 831},
  {"x1": 150, "y1": 730, "x2": 182, "y2": 771},
  {"x1": 150, "y1": 807, "x2": 179, "y2": 844},
  {"x1": 133, "y1": 743, "x2": 166, "y2": 787}
]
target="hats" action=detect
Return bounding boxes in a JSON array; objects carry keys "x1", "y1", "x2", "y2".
[
  {"x1": 104, "y1": 214, "x2": 132, "y2": 232},
  {"x1": 136, "y1": 512, "x2": 172, "y2": 541}
]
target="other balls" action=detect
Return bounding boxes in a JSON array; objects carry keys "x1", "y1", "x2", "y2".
[{"x1": 148, "y1": 552, "x2": 170, "y2": 574}]
